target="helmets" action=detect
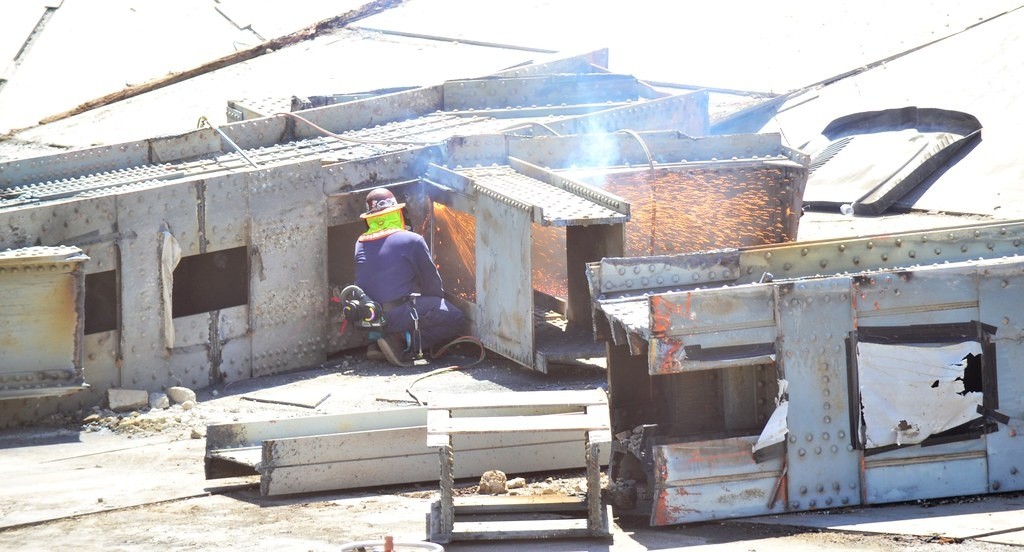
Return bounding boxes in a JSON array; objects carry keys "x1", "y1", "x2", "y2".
[{"x1": 359, "y1": 187, "x2": 407, "y2": 218}]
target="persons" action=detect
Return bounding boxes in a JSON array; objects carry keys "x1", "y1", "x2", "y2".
[{"x1": 350, "y1": 186, "x2": 466, "y2": 368}]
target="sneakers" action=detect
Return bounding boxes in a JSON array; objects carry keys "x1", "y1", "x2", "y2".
[{"x1": 366, "y1": 334, "x2": 428, "y2": 366}]
